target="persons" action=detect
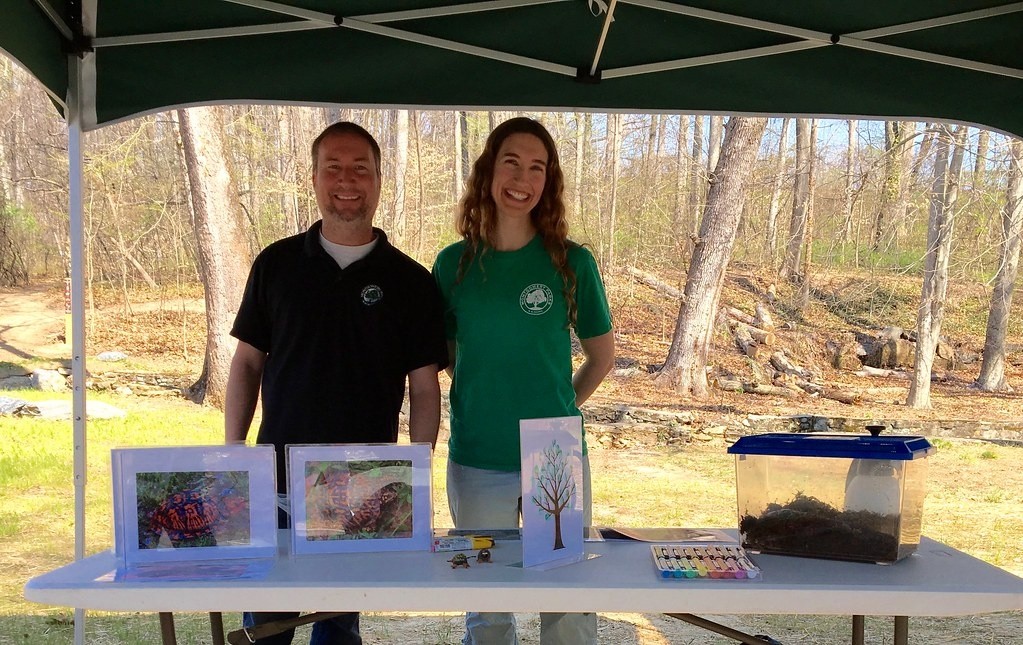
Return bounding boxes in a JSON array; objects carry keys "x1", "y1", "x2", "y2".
[
  {"x1": 430, "y1": 115, "x2": 617, "y2": 645},
  {"x1": 223, "y1": 119, "x2": 450, "y2": 645}
]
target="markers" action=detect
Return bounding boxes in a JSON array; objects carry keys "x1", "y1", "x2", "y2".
[{"x1": 651, "y1": 544, "x2": 758, "y2": 580}]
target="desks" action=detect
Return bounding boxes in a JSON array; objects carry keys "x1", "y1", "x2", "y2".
[{"x1": 24, "y1": 526, "x2": 1023, "y2": 645}]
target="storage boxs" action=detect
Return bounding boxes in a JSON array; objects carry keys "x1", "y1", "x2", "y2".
[{"x1": 727, "y1": 432, "x2": 937, "y2": 566}]
